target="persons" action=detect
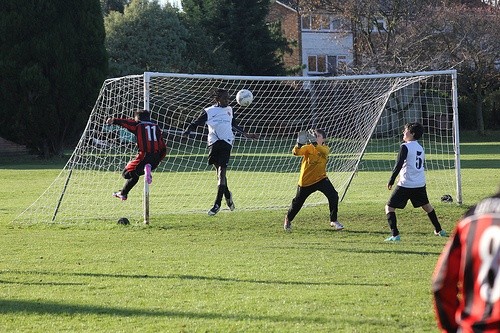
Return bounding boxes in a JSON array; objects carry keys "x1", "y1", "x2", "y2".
[
  {"x1": 430, "y1": 192, "x2": 500, "y2": 333},
  {"x1": 283, "y1": 128, "x2": 345, "y2": 233},
  {"x1": 383, "y1": 122, "x2": 448, "y2": 243},
  {"x1": 181, "y1": 89, "x2": 258, "y2": 216},
  {"x1": 105, "y1": 109, "x2": 167, "y2": 200}
]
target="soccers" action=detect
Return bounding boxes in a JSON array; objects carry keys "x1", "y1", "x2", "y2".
[{"x1": 235, "y1": 89, "x2": 254, "y2": 107}]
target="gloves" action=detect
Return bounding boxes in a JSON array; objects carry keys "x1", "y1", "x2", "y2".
[
  {"x1": 307, "y1": 128, "x2": 318, "y2": 143},
  {"x1": 297, "y1": 130, "x2": 308, "y2": 145}
]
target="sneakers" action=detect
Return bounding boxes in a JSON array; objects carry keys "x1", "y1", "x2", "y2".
[
  {"x1": 226, "y1": 191, "x2": 234, "y2": 207},
  {"x1": 144, "y1": 164, "x2": 152, "y2": 183},
  {"x1": 330, "y1": 221, "x2": 343, "y2": 230},
  {"x1": 284, "y1": 215, "x2": 291, "y2": 231},
  {"x1": 434, "y1": 229, "x2": 446, "y2": 237},
  {"x1": 112, "y1": 191, "x2": 127, "y2": 200},
  {"x1": 208, "y1": 204, "x2": 220, "y2": 215},
  {"x1": 384, "y1": 234, "x2": 400, "y2": 242}
]
